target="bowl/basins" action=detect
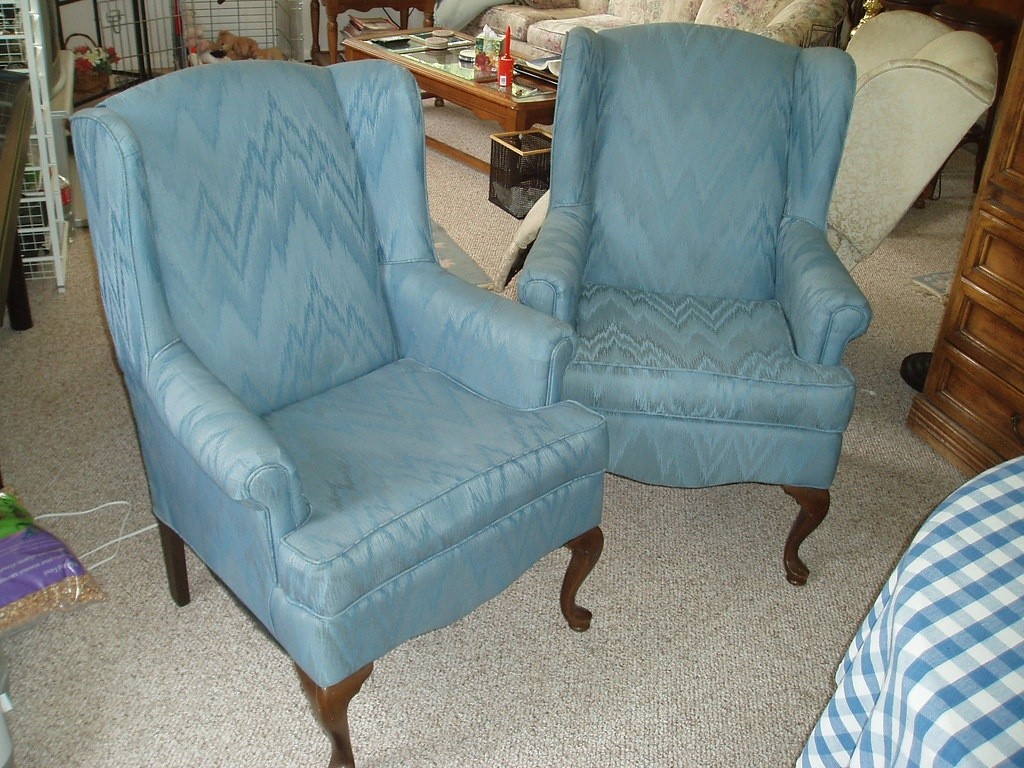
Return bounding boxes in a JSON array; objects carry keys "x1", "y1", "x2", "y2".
[{"x1": 426, "y1": 37, "x2": 448, "y2": 49}]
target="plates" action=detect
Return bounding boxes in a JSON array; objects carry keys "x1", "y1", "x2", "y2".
[{"x1": 432, "y1": 30, "x2": 454, "y2": 37}]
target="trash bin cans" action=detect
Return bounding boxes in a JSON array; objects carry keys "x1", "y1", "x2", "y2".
[{"x1": 488, "y1": 129, "x2": 553, "y2": 221}]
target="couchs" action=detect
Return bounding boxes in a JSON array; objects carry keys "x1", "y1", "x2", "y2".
[{"x1": 460, "y1": 1, "x2": 848, "y2": 66}]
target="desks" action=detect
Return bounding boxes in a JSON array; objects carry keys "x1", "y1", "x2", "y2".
[{"x1": 310, "y1": 0, "x2": 436, "y2": 66}]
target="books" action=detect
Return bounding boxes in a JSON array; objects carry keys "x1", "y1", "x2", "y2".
[
  {"x1": 513, "y1": 56, "x2": 561, "y2": 92},
  {"x1": 340, "y1": 13, "x2": 399, "y2": 39}
]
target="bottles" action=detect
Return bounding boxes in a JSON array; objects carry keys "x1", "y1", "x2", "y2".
[{"x1": 58, "y1": 174, "x2": 77, "y2": 244}]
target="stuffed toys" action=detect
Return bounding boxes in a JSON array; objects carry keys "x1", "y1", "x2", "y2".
[{"x1": 183, "y1": 25, "x2": 265, "y2": 67}]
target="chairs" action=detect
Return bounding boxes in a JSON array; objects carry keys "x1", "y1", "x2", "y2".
[
  {"x1": 74, "y1": 58, "x2": 607, "y2": 768},
  {"x1": 495, "y1": 10, "x2": 999, "y2": 287},
  {"x1": 519, "y1": 21, "x2": 879, "y2": 592}
]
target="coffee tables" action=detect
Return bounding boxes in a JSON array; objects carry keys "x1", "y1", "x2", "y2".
[{"x1": 340, "y1": 26, "x2": 559, "y2": 173}]
